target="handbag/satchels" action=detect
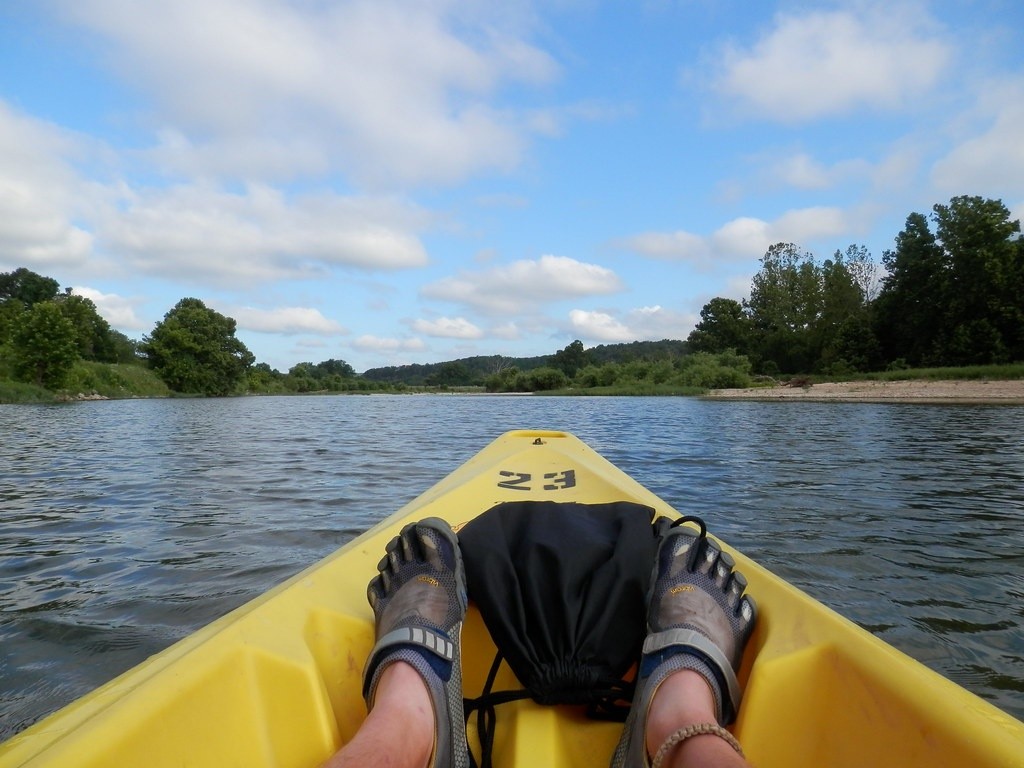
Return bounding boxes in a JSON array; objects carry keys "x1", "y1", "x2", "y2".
[{"x1": 456, "y1": 500, "x2": 667, "y2": 708}]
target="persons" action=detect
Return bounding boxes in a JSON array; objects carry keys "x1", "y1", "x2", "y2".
[{"x1": 317, "y1": 517, "x2": 758, "y2": 768}]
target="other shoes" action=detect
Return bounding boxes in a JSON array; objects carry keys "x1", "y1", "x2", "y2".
[
  {"x1": 611, "y1": 527, "x2": 757, "y2": 768},
  {"x1": 362, "y1": 518, "x2": 476, "y2": 768}
]
